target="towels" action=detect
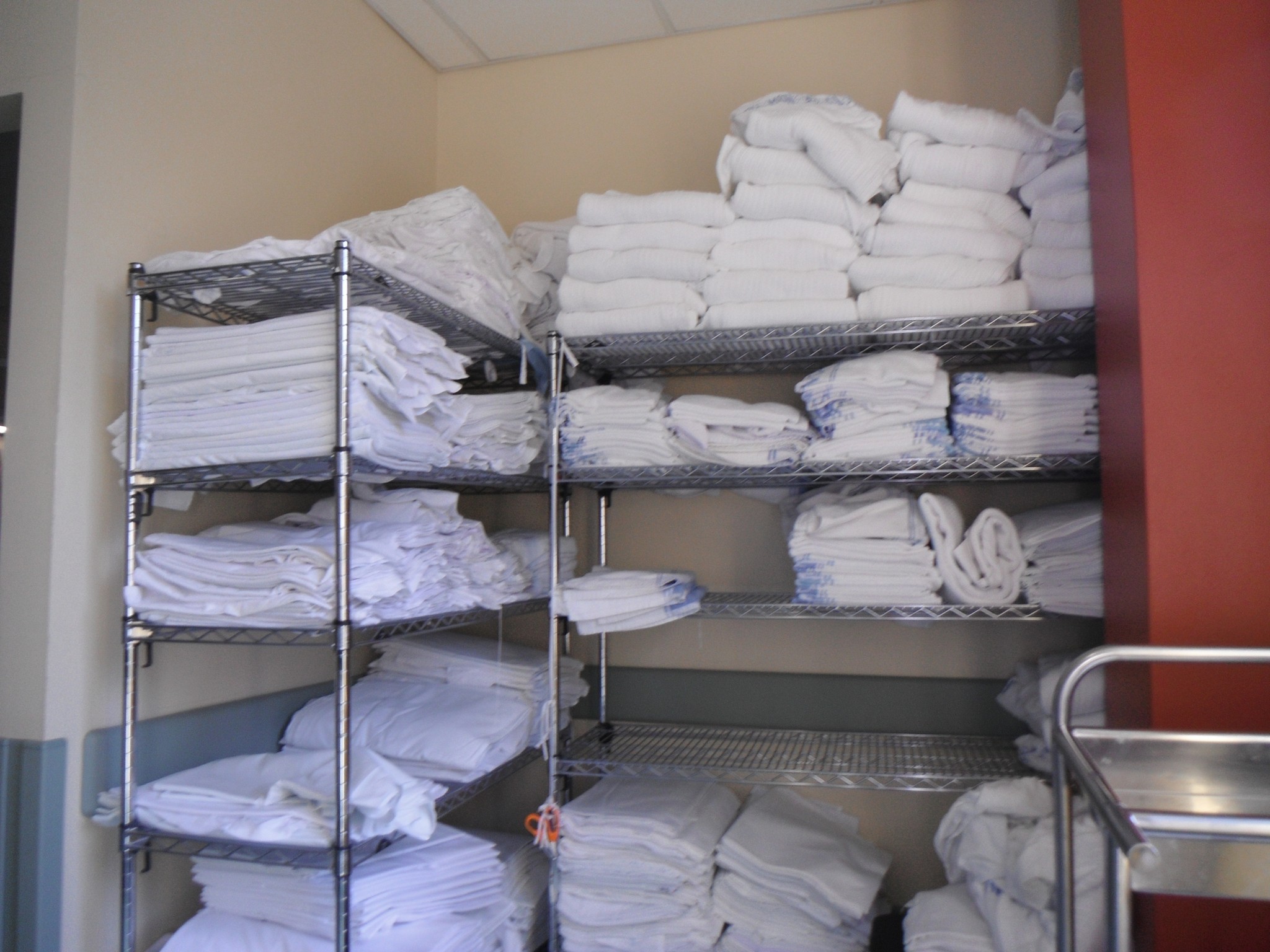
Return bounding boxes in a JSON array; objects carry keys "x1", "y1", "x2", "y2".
[{"x1": 107, "y1": 69, "x2": 1112, "y2": 952}]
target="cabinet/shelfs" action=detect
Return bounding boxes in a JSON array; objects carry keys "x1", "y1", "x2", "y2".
[
  {"x1": 540, "y1": 305, "x2": 1103, "y2": 951},
  {"x1": 116, "y1": 238, "x2": 610, "y2": 952}
]
